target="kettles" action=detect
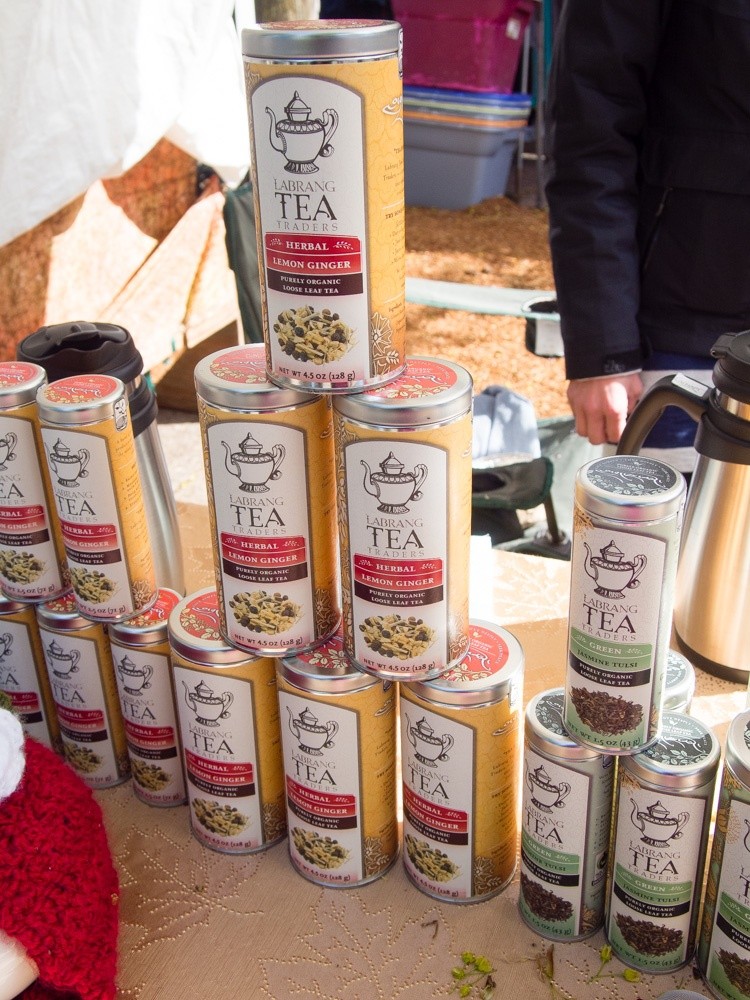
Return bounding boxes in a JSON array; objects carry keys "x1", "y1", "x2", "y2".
[{"x1": 615, "y1": 328, "x2": 750, "y2": 685}]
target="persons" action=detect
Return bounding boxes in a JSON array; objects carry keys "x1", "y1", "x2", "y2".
[{"x1": 543, "y1": 0, "x2": 750, "y2": 485}]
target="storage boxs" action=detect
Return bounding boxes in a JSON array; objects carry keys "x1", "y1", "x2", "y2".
[{"x1": 393, "y1": 0, "x2": 540, "y2": 212}]
